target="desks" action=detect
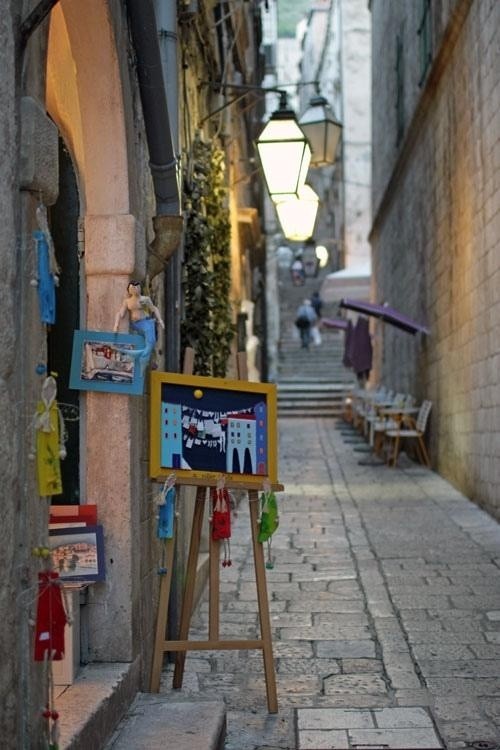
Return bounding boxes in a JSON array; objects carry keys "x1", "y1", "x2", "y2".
[{"x1": 51, "y1": 583, "x2": 87, "y2": 685}]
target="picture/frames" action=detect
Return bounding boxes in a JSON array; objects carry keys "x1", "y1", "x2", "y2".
[
  {"x1": 49, "y1": 525, "x2": 105, "y2": 580},
  {"x1": 69, "y1": 329, "x2": 144, "y2": 394}
]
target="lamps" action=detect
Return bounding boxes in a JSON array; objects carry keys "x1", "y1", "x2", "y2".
[{"x1": 197, "y1": 78, "x2": 342, "y2": 242}]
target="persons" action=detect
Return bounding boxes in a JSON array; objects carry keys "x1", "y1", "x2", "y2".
[
  {"x1": 295, "y1": 299, "x2": 318, "y2": 349},
  {"x1": 291, "y1": 254, "x2": 305, "y2": 287},
  {"x1": 114, "y1": 280, "x2": 165, "y2": 359},
  {"x1": 307, "y1": 290, "x2": 324, "y2": 343}
]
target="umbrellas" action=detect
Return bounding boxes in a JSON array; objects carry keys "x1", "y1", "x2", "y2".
[
  {"x1": 337, "y1": 297, "x2": 429, "y2": 341},
  {"x1": 343, "y1": 315, "x2": 373, "y2": 387},
  {"x1": 318, "y1": 318, "x2": 349, "y2": 332}
]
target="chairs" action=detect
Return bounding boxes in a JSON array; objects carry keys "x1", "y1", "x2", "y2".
[{"x1": 350, "y1": 384, "x2": 432, "y2": 470}]
